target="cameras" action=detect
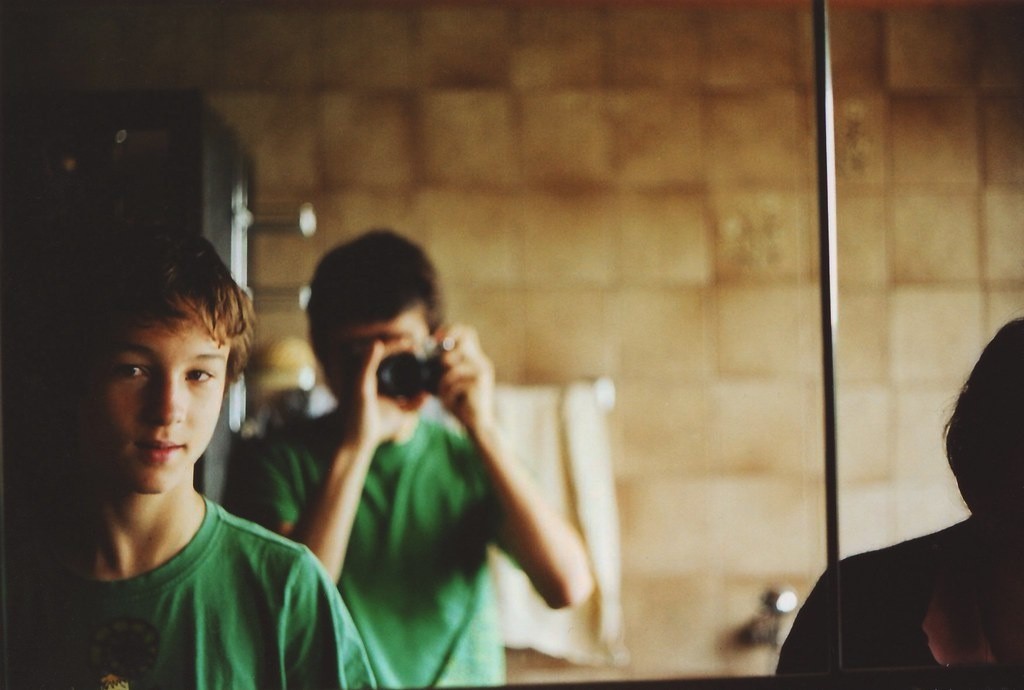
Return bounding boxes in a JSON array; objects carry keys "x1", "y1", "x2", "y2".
[{"x1": 377, "y1": 349, "x2": 451, "y2": 401}]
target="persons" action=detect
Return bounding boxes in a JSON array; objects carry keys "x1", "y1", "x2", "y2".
[
  {"x1": 775, "y1": 318, "x2": 1024, "y2": 690},
  {"x1": 0, "y1": 229, "x2": 379, "y2": 690},
  {"x1": 240, "y1": 228, "x2": 594, "y2": 690}
]
